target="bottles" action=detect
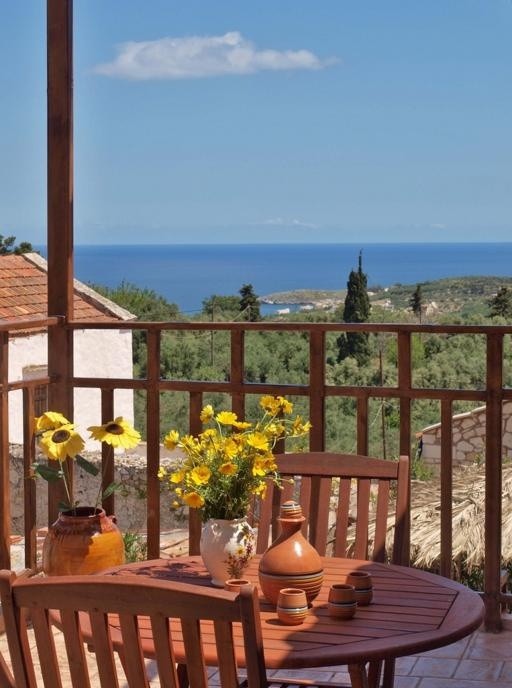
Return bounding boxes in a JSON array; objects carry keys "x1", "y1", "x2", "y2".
[{"x1": 258, "y1": 501, "x2": 324, "y2": 605}]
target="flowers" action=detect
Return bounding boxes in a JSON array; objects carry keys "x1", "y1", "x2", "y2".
[
  {"x1": 34, "y1": 411, "x2": 142, "y2": 513},
  {"x1": 157, "y1": 395, "x2": 312, "y2": 526}
]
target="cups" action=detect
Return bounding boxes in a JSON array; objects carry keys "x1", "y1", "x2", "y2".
[
  {"x1": 345, "y1": 572, "x2": 373, "y2": 607},
  {"x1": 328, "y1": 584, "x2": 357, "y2": 620},
  {"x1": 277, "y1": 588, "x2": 309, "y2": 625},
  {"x1": 224, "y1": 579, "x2": 251, "y2": 593}
]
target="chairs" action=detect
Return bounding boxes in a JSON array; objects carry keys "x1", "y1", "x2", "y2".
[
  {"x1": 178, "y1": 452, "x2": 409, "y2": 688},
  {"x1": 0, "y1": 570, "x2": 267, "y2": 688}
]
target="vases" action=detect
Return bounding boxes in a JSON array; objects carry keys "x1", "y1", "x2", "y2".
[
  {"x1": 200, "y1": 517, "x2": 257, "y2": 588},
  {"x1": 41, "y1": 507, "x2": 125, "y2": 575}
]
